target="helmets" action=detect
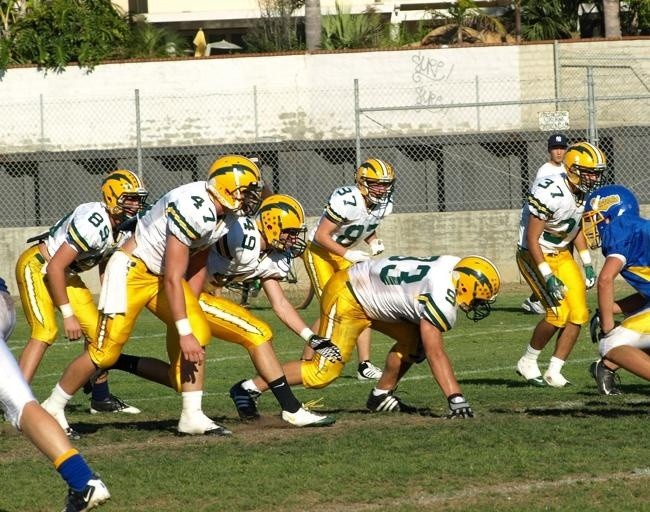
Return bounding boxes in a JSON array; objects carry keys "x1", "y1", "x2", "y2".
[
  {"x1": 100, "y1": 170, "x2": 149, "y2": 221},
  {"x1": 354, "y1": 158, "x2": 396, "y2": 205},
  {"x1": 206, "y1": 154, "x2": 264, "y2": 217},
  {"x1": 582, "y1": 184, "x2": 640, "y2": 251},
  {"x1": 562, "y1": 142, "x2": 607, "y2": 193},
  {"x1": 255, "y1": 194, "x2": 308, "y2": 259},
  {"x1": 451, "y1": 255, "x2": 501, "y2": 322}
]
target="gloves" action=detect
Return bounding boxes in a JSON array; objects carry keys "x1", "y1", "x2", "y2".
[
  {"x1": 343, "y1": 249, "x2": 370, "y2": 263},
  {"x1": 583, "y1": 262, "x2": 597, "y2": 289},
  {"x1": 544, "y1": 273, "x2": 568, "y2": 301},
  {"x1": 590, "y1": 308, "x2": 602, "y2": 345},
  {"x1": 446, "y1": 393, "x2": 475, "y2": 419},
  {"x1": 308, "y1": 335, "x2": 342, "y2": 364},
  {"x1": 369, "y1": 238, "x2": 385, "y2": 256}
]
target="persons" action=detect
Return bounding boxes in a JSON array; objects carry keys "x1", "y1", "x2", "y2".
[
  {"x1": 590, "y1": 292, "x2": 650, "y2": 394},
  {"x1": 305, "y1": 159, "x2": 398, "y2": 381},
  {"x1": 80, "y1": 193, "x2": 346, "y2": 427},
  {"x1": 520, "y1": 135, "x2": 575, "y2": 314},
  {"x1": 229, "y1": 255, "x2": 501, "y2": 425},
  {"x1": 17, "y1": 169, "x2": 148, "y2": 413},
  {"x1": 583, "y1": 184, "x2": 650, "y2": 383},
  {"x1": 0, "y1": 278, "x2": 110, "y2": 511},
  {"x1": 515, "y1": 142, "x2": 607, "y2": 389},
  {"x1": 38, "y1": 154, "x2": 262, "y2": 441}
]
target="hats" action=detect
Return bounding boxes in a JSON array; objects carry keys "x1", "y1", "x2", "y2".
[{"x1": 547, "y1": 134, "x2": 568, "y2": 148}]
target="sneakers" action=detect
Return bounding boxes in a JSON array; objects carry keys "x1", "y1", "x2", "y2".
[
  {"x1": 543, "y1": 370, "x2": 572, "y2": 389},
  {"x1": 228, "y1": 379, "x2": 260, "y2": 421},
  {"x1": 366, "y1": 387, "x2": 412, "y2": 414},
  {"x1": 89, "y1": 394, "x2": 141, "y2": 415},
  {"x1": 39, "y1": 398, "x2": 81, "y2": 441},
  {"x1": 515, "y1": 356, "x2": 546, "y2": 387},
  {"x1": 281, "y1": 402, "x2": 336, "y2": 428},
  {"x1": 176, "y1": 410, "x2": 232, "y2": 437},
  {"x1": 357, "y1": 362, "x2": 383, "y2": 380},
  {"x1": 521, "y1": 296, "x2": 546, "y2": 314},
  {"x1": 59, "y1": 473, "x2": 110, "y2": 512},
  {"x1": 589, "y1": 359, "x2": 622, "y2": 397}
]
